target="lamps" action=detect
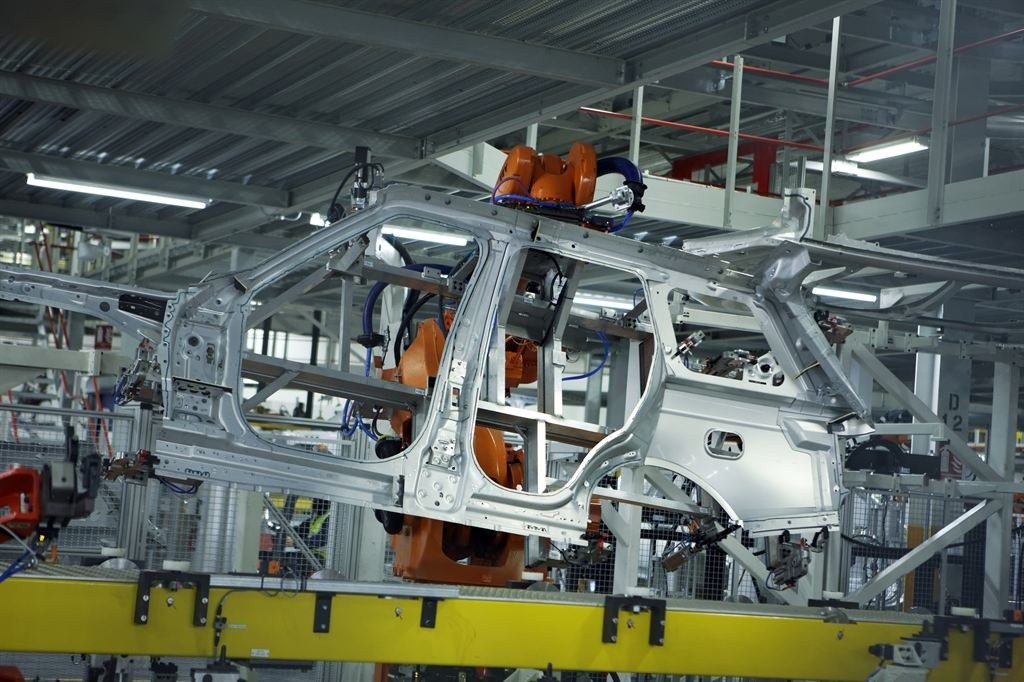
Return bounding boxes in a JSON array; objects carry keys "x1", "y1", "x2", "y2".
[{"x1": 25, "y1": 134, "x2": 932, "y2": 309}]
